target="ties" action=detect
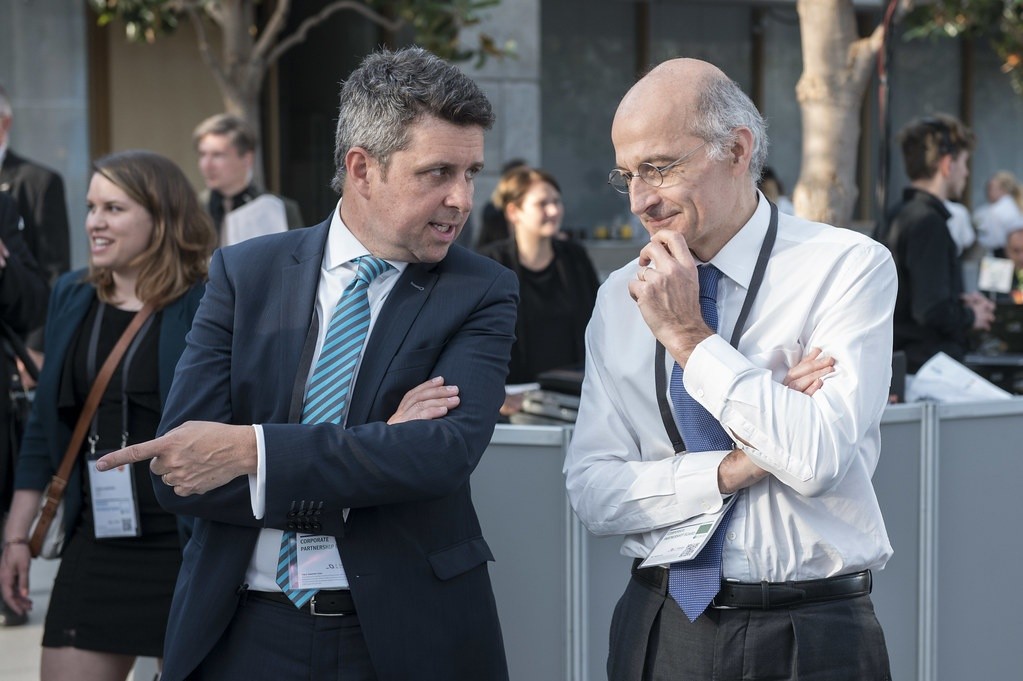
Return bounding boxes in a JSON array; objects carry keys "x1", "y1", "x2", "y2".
[
  {"x1": 275, "y1": 255, "x2": 395, "y2": 608},
  {"x1": 667, "y1": 263, "x2": 739, "y2": 623}
]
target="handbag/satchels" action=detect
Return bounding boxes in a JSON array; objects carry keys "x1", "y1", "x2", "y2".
[{"x1": 29, "y1": 477, "x2": 66, "y2": 560}]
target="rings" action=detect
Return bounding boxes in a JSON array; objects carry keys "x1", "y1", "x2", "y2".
[
  {"x1": 641, "y1": 267, "x2": 652, "y2": 281},
  {"x1": 163, "y1": 474, "x2": 172, "y2": 486}
]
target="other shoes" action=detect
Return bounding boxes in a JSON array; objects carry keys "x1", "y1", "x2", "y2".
[{"x1": 5, "y1": 613, "x2": 28, "y2": 627}]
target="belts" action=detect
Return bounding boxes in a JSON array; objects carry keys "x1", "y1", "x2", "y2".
[
  {"x1": 245, "y1": 590, "x2": 357, "y2": 616},
  {"x1": 631, "y1": 558, "x2": 870, "y2": 610}
]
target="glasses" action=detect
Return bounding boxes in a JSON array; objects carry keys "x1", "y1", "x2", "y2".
[{"x1": 607, "y1": 126, "x2": 737, "y2": 194}]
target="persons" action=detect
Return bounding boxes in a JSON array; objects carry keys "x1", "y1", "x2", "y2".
[
  {"x1": 0, "y1": 93, "x2": 73, "y2": 624},
  {"x1": 759, "y1": 165, "x2": 796, "y2": 214},
  {"x1": 475, "y1": 154, "x2": 601, "y2": 414},
  {"x1": 560, "y1": 56, "x2": 898, "y2": 681},
  {"x1": 96, "y1": 46, "x2": 521, "y2": 681},
  {"x1": 0, "y1": 152, "x2": 217, "y2": 681},
  {"x1": 945, "y1": 171, "x2": 1023, "y2": 348},
  {"x1": 193, "y1": 111, "x2": 305, "y2": 249},
  {"x1": 869, "y1": 112, "x2": 995, "y2": 376}
]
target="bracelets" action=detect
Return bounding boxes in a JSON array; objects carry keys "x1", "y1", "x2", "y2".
[{"x1": 5, "y1": 538, "x2": 27, "y2": 547}]
